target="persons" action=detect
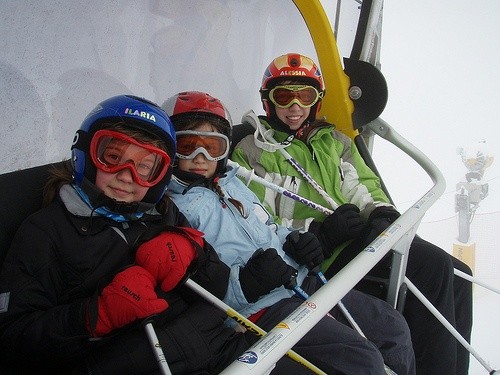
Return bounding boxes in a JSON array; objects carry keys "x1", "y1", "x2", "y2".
[
  {"x1": 232, "y1": 53, "x2": 474, "y2": 375},
  {"x1": 0, "y1": 95, "x2": 344, "y2": 375},
  {"x1": 155, "y1": 90, "x2": 417, "y2": 375}
]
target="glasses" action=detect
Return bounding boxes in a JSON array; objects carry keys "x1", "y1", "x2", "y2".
[
  {"x1": 175, "y1": 129, "x2": 231, "y2": 161},
  {"x1": 88, "y1": 129, "x2": 173, "y2": 187},
  {"x1": 267, "y1": 84, "x2": 320, "y2": 109}
]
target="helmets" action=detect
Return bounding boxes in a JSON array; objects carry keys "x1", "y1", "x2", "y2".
[
  {"x1": 70, "y1": 94, "x2": 178, "y2": 224},
  {"x1": 163, "y1": 91, "x2": 233, "y2": 177},
  {"x1": 258, "y1": 54, "x2": 325, "y2": 121}
]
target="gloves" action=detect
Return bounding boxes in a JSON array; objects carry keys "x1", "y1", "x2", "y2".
[
  {"x1": 308, "y1": 204, "x2": 368, "y2": 260},
  {"x1": 364, "y1": 206, "x2": 401, "y2": 266},
  {"x1": 284, "y1": 229, "x2": 324, "y2": 268},
  {"x1": 240, "y1": 247, "x2": 291, "y2": 304},
  {"x1": 79, "y1": 264, "x2": 169, "y2": 339},
  {"x1": 136, "y1": 225, "x2": 206, "y2": 293}
]
